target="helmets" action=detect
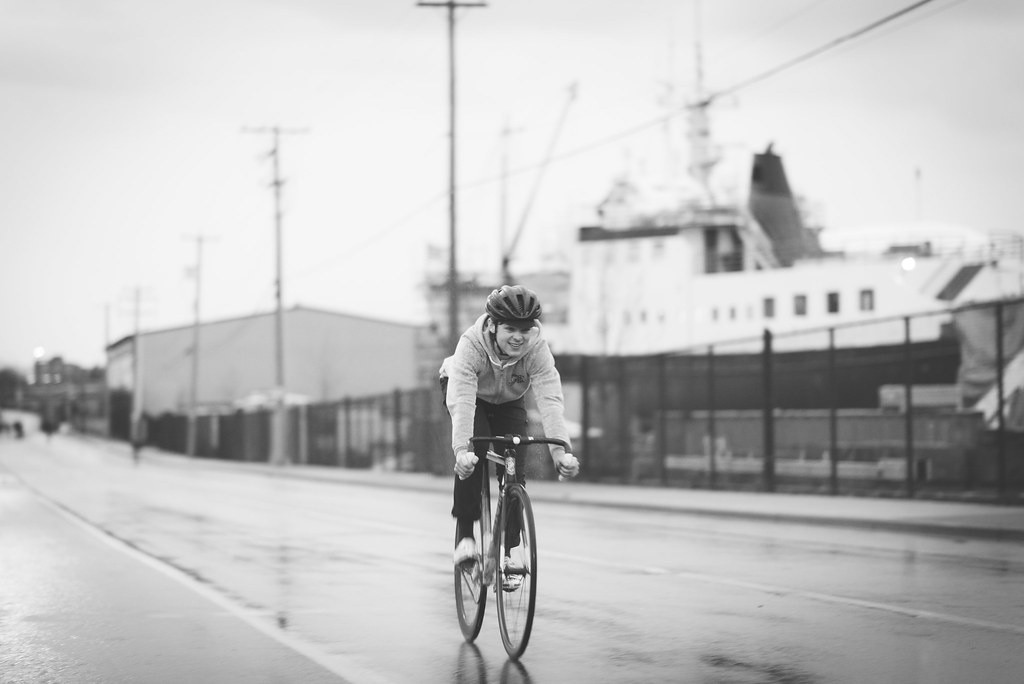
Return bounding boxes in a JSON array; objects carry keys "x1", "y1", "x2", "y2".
[{"x1": 485, "y1": 284, "x2": 542, "y2": 323}]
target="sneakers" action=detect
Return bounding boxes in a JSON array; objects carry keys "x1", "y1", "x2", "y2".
[
  {"x1": 454, "y1": 537, "x2": 477, "y2": 571},
  {"x1": 502, "y1": 555, "x2": 525, "y2": 593}
]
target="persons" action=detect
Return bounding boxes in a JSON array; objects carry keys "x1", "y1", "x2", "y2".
[{"x1": 437, "y1": 285, "x2": 580, "y2": 592}]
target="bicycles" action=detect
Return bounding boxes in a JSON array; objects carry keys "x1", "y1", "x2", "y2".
[{"x1": 453, "y1": 433, "x2": 573, "y2": 661}]
